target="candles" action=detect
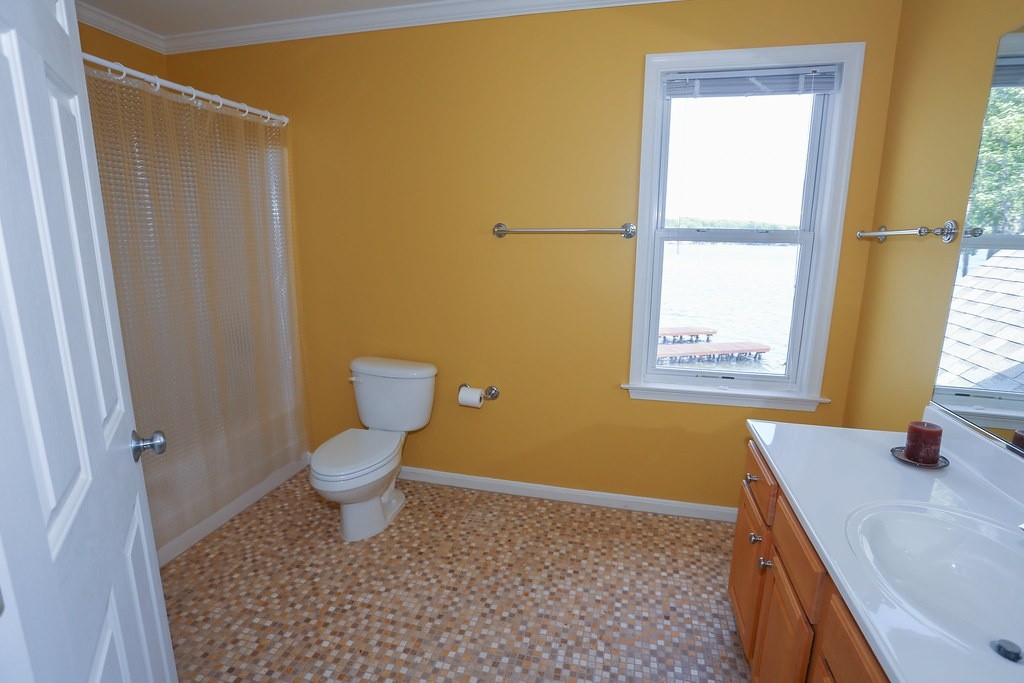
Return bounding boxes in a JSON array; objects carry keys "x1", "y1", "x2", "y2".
[{"x1": 905, "y1": 421, "x2": 942, "y2": 464}]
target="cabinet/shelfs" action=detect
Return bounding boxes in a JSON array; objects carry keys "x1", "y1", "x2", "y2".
[{"x1": 727, "y1": 438, "x2": 889, "y2": 682}]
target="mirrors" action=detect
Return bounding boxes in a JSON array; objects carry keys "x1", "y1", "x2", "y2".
[{"x1": 931, "y1": 32, "x2": 1023, "y2": 464}]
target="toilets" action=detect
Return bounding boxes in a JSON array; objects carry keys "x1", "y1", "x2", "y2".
[{"x1": 307, "y1": 354, "x2": 440, "y2": 544}]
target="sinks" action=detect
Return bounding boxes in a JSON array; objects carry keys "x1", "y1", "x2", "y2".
[{"x1": 844, "y1": 503, "x2": 1023, "y2": 677}]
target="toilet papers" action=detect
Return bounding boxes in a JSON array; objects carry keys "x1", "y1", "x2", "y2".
[{"x1": 457, "y1": 387, "x2": 486, "y2": 409}]
